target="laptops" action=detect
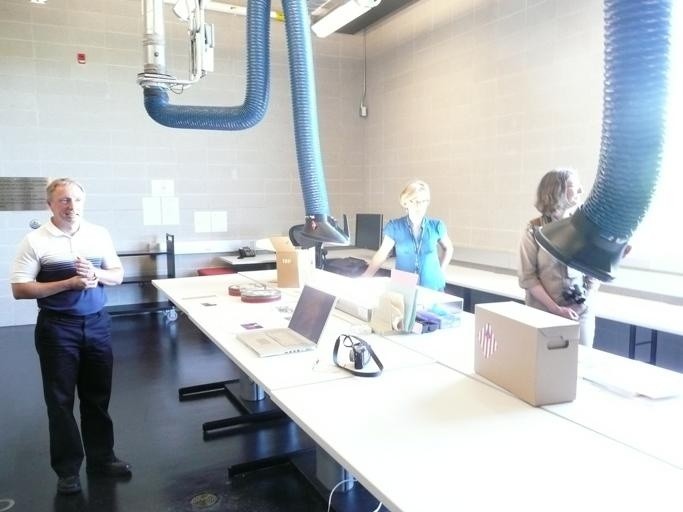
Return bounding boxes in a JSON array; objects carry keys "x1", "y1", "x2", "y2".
[{"x1": 235, "y1": 283, "x2": 339, "y2": 359}]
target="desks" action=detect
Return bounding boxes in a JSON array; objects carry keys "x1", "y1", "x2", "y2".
[
  {"x1": 221, "y1": 248, "x2": 391, "y2": 265},
  {"x1": 245, "y1": 261, "x2": 464, "y2": 321},
  {"x1": 142, "y1": 267, "x2": 683, "y2": 512},
  {"x1": 352, "y1": 252, "x2": 683, "y2": 336}
]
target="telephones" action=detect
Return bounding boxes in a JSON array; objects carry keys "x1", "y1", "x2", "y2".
[{"x1": 237, "y1": 247, "x2": 255, "y2": 259}]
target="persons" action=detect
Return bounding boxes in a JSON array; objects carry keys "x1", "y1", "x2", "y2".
[
  {"x1": 518, "y1": 168, "x2": 601, "y2": 348},
  {"x1": 11, "y1": 178, "x2": 130, "y2": 494},
  {"x1": 359, "y1": 180, "x2": 454, "y2": 292}
]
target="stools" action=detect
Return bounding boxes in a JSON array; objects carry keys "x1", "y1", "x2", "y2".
[{"x1": 197, "y1": 267, "x2": 237, "y2": 275}]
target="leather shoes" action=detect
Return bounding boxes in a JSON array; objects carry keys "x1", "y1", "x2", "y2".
[
  {"x1": 57, "y1": 472, "x2": 81, "y2": 493},
  {"x1": 86, "y1": 458, "x2": 132, "y2": 476}
]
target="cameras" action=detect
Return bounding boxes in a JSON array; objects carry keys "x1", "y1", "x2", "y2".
[
  {"x1": 349, "y1": 343, "x2": 373, "y2": 369},
  {"x1": 563, "y1": 283, "x2": 587, "y2": 306}
]
